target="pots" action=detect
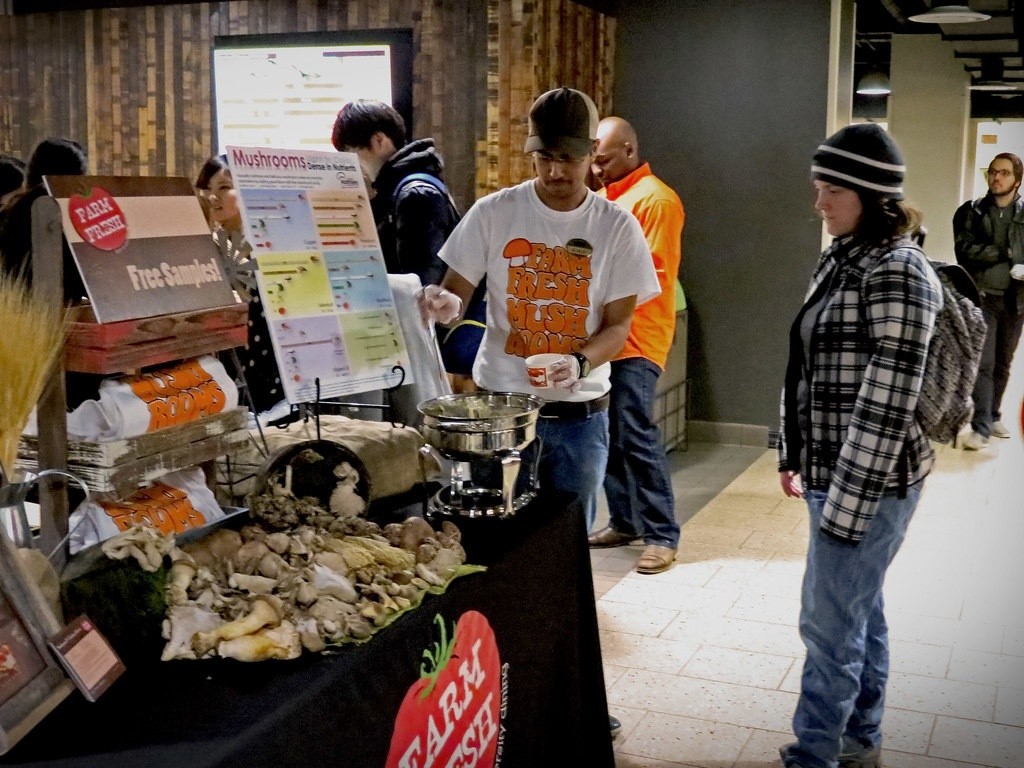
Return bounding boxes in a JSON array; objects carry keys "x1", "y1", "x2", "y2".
[{"x1": 418, "y1": 390, "x2": 547, "y2": 462}]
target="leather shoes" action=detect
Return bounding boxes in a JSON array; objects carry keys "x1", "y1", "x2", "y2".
[
  {"x1": 635, "y1": 545, "x2": 679, "y2": 573},
  {"x1": 588, "y1": 527, "x2": 644, "y2": 548}
]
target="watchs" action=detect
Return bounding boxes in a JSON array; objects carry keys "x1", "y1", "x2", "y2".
[{"x1": 571, "y1": 352, "x2": 592, "y2": 380}]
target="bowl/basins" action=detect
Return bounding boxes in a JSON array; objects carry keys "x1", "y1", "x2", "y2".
[{"x1": 525, "y1": 353, "x2": 564, "y2": 390}]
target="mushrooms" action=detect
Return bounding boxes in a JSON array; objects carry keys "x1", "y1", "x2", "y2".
[{"x1": 155, "y1": 489, "x2": 465, "y2": 665}]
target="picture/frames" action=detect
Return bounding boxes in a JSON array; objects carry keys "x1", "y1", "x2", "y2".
[{"x1": 0, "y1": 522, "x2": 77, "y2": 756}]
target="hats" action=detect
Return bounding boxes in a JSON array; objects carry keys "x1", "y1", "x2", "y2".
[
  {"x1": 523, "y1": 86, "x2": 599, "y2": 157},
  {"x1": 811, "y1": 124, "x2": 905, "y2": 201}
]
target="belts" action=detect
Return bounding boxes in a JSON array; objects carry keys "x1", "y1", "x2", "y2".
[{"x1": 539, "y1": 394, "x2": 612, "y2": 417}]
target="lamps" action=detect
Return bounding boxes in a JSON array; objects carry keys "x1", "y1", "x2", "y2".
[{"x1": 856, "y1": 73, "x2": 891, "y2": 96}]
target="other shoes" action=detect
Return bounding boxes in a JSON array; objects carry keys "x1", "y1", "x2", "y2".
[{"x1": 779, "y1": 728, "x2": 882, "y2": 768}]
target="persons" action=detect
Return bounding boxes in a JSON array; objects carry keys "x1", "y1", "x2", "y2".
[
  {"x1": 194, "y1": 155, "x2": 285, "y2": 419},
  {"x1": 775, "y1": 123, "x2": 943, "y2": 768},
  {"x1": 0, "y1": 137, "x2": 86, "y2": 310},
  {"x1": 424, "y1": 86, "x2": 663, "y2": 739},
  {"x1": 588, "y1": 116, "x2": 684, "y2": 575},
  {"x1": 953, "y1": 153, "x2": 1024, "y2": 450},
  {"x1": 331, "y1": 97, "x2": 486, "y2": 367}
]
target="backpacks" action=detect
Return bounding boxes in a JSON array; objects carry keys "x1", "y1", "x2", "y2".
[{"x1": 860, "y1": 239, "x2": 988, "y2": 445}]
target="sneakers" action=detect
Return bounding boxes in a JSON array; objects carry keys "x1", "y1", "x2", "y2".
[
  {"x1": 991, "y1": 420, "x2": 1011, "y2": 438},
  {"x1": 962, "y1": 433, "x2": 988, "y2": 450}
]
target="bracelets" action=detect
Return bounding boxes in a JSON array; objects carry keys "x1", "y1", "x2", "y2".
[{"x1": 454, "y1": 295, "x2": 465, "y2": 320}]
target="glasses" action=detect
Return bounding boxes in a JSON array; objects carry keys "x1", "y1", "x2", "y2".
[
  {"x1": 532, "y1": 154, "x2": 586, "y2": 166},
  {"x1": 988, "y1": 169, "x2": 1014, "y2": 177}
]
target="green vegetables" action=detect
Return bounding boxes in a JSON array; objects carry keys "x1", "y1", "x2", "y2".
[{"x1": 64, "y1": 543, "x2": 174, "y2": 621}]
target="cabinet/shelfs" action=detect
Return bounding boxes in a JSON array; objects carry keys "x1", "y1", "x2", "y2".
[{"x1": 6, "y1": 194, "x2": 253, "y2": 583}]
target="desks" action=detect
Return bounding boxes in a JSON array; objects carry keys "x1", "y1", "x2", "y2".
[{"x1": 0, "y1": 491, "x2": 616, "y2": 768}]
